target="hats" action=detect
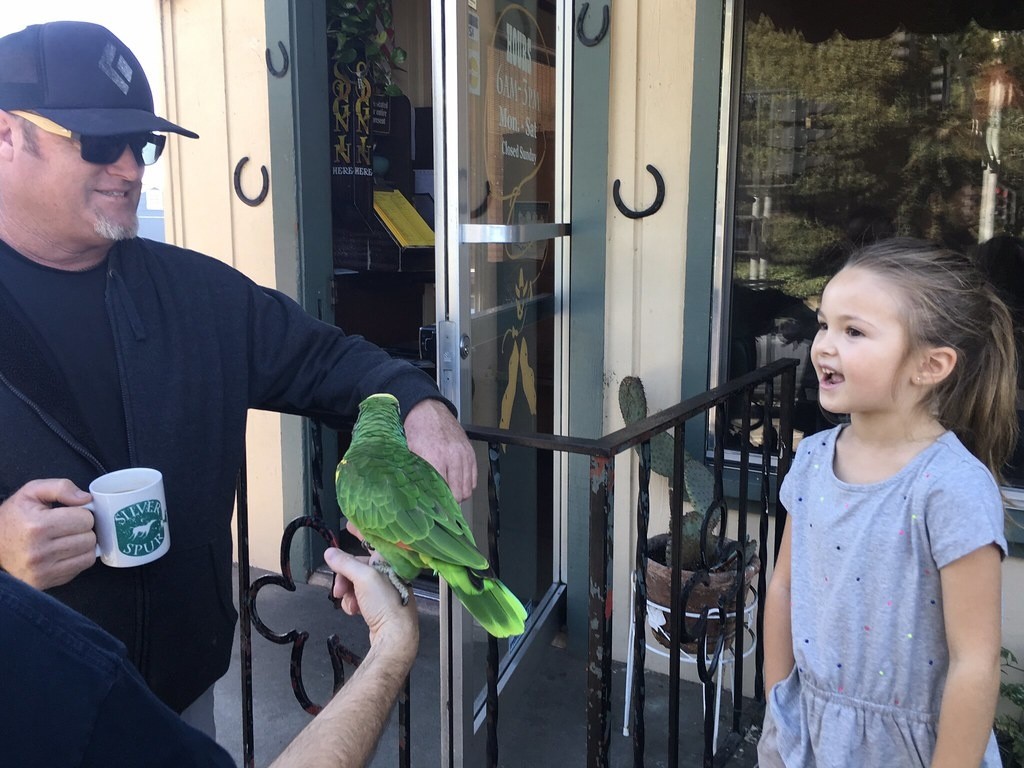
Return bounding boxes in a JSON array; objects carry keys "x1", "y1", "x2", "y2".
[{"x1": 0, "y1": 22, "x2": 199, "y2": 138}]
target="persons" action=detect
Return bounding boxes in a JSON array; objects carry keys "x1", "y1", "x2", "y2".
[
  {"x1": 0, "y1": 517, "x2": 418, "y2": 768},
  {"x1": 0, "y1": 22, "x2": 479, "y2": 740},
  {"x1": 757, "y1": 237, "x2": 1018, "y2": 768}
]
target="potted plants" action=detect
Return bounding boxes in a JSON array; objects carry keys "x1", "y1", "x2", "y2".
[{"x1": 618, "y1": 375, "x2": 762, "y2": 655}]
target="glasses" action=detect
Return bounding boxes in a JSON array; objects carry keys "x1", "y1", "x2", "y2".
[{"x1": 7, "y1": 110, "x2": 167, "y2": 166}]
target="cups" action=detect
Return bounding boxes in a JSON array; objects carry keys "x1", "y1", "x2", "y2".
[{"x1": 68, "y1": 467, "x2": 172, "y2": 568}]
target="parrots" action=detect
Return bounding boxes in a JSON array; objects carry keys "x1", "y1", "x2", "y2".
[{"x1": 335, "y1": 393, "x2": 529, "y2": 639}]
